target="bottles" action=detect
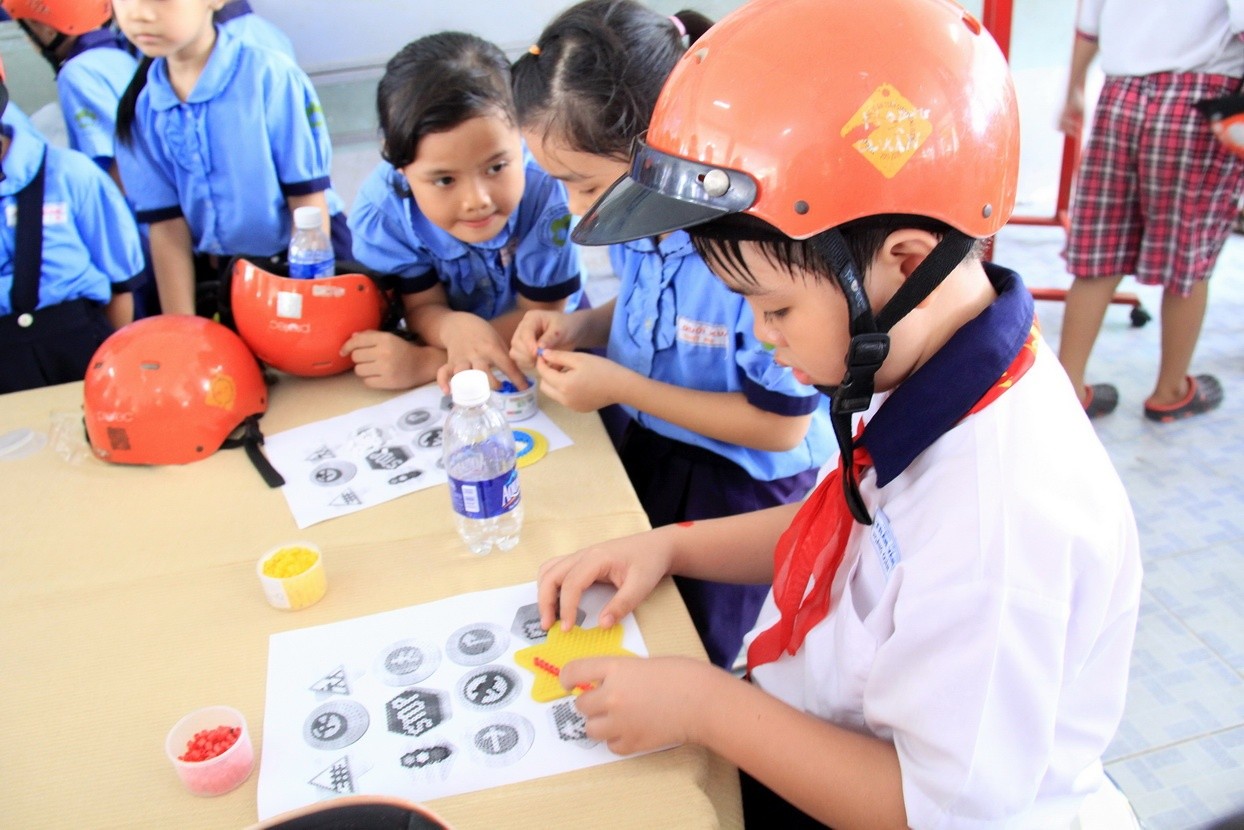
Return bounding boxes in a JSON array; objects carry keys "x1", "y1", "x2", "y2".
[
  {"x1": 439, "y1": 369, "x2": 525, "y2": 557},
  {"x1": 287, "y1": 205, "x2": 336, "y2": 280}
]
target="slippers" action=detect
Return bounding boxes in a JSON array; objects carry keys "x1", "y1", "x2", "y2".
[
  {"x1": 1145, "y1": 375, "x2": 1222, "y2": 419},
  {"x1": 1069, "y1": 385, "x2": 1118, "y2": 414}
]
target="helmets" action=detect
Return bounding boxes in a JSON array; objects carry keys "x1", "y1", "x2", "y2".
[
  {"x1": 84, "y1": 314, "x2": 266, "y2": 464},
  {"x1": 1195, "y1": 95, "x2": 1244, "y2": 157},
  {"x1": 1, "y1": 0, "x2": 113, "y2": 34},
  {"x1": 570, "y1": 0, "x2": 1020, "y2": 246},
  {"x1": 232, "y1": 257, "x2": 380, "y2": 377}
]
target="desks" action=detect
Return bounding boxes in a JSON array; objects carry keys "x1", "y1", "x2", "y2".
[{"x1": 1, "y1": 363, "x2": 743, "y2": 830}]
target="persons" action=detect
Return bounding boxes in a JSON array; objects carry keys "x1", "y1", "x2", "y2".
[
  {"x1": 0, "y1": 0, "x2": 352, "y2": 394},
  {"x1": 336, "y1": 34, "x2": 585, "y2": 390},
  {"x1": 1058, "y1": 0, "x2": 1244, "y2": 422},
  {"x1": 507, "y1": 1, "x2": 840, "y2": 678},
  {"x1": 539, "y1": 0, "x2": 1144, "y2": 830}
]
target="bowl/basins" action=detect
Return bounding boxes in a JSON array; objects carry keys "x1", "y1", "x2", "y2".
[
  {"x1": 488, "y1": 370, "x2": 538, "y2": 423},
  {"x1": 165, "y1": 705, "x2": 256, "y2": 798},
  {"x1": 253, "y1": 542, "x2": 329, "y2": 612}
]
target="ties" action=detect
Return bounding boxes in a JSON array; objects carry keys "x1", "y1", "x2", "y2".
[{"x1": 747, "y1": 308, "x2": 1042, "y2": 675}]
